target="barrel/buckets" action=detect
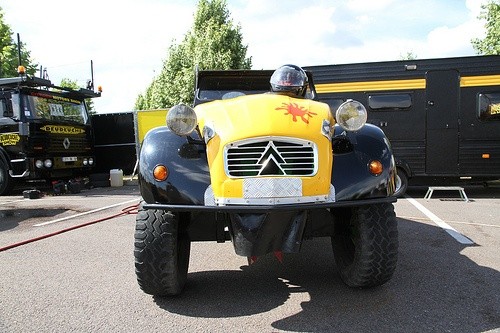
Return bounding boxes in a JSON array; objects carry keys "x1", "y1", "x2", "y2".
[{"x1": 109, "y1": 168, "x2": 123, "y2": 187}]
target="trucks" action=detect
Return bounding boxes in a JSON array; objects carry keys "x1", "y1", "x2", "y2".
[
  {"x1": 0, "y1": 65, "x2": 103, "y2": 197},
  {"x1": 300, "y1": 54, "x2": 500, "y2": 198}
]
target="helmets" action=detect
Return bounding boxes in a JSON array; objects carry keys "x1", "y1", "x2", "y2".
[{"x1": 270, "y1": 64, "x2": 308, "y2": 97}]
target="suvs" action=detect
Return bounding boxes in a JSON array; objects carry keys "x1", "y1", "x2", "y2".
[{"x1": 130, "y1": 64, "x2": 400, "y2": 298}]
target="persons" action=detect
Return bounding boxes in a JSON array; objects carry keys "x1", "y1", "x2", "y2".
[{"x1": 269, "y1": 64, "x2": 308, "y2": 99}]
